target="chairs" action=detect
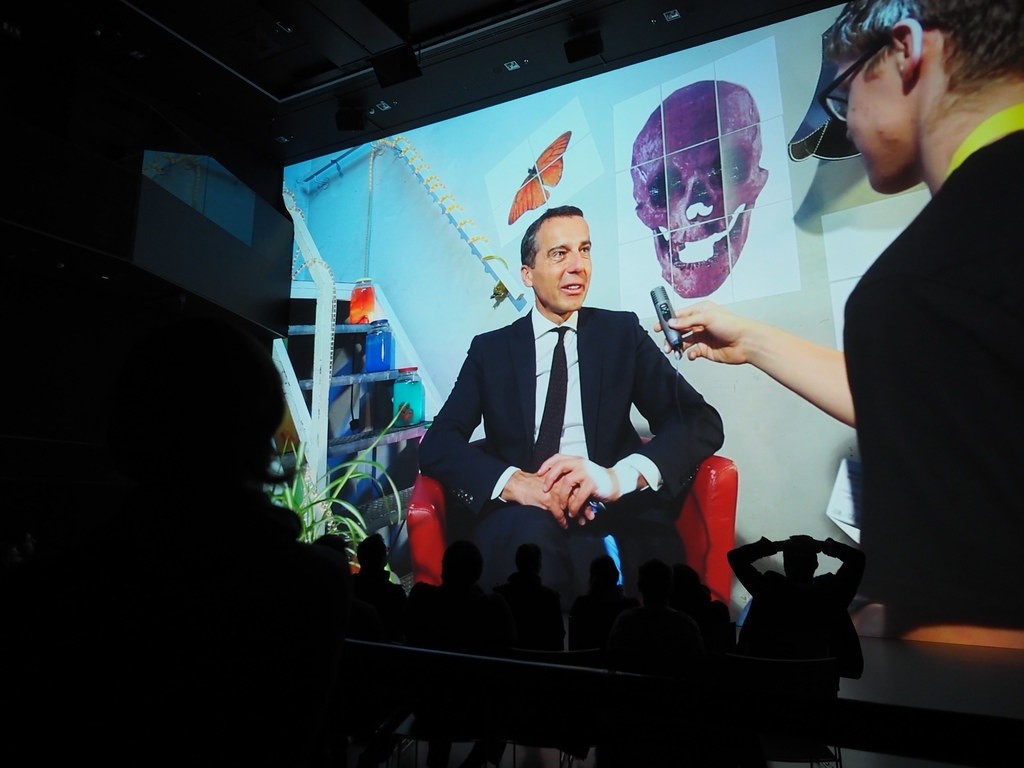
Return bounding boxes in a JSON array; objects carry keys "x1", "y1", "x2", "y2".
[{"x1": 726, "y1": 652, "x2": 843, "y2": 768}]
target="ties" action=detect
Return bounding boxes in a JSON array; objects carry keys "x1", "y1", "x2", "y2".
[{"x1": 533, "y1": 330, "x2": 570, "y2": 473}]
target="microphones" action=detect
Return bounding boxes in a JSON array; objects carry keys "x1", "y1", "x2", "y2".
[{"x1": 651, "y1": 285, "x2": 683, "y2": 351}]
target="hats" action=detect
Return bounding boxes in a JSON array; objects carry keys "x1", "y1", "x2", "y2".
[{"x1": 787, "y1": 43, "x2": 867, "y2": 163}]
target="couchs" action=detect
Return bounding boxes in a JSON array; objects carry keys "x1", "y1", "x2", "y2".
[{"x1": 405, "y1": 437, "x2": 740, "y2": 604}]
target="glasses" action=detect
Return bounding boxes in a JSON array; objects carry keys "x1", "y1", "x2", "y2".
[{"x1": 817, "y1": 17, "x2": 975, "y2": 124}]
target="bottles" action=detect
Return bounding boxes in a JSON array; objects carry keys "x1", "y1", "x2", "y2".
[
  {"x1": 366, "y1": 319, "x2": 391, "y2": 372},
  {"x1": 349, "y1": 277, "x2": 375, "y2": 324},
  {"x1": 393, "y1": 367, "x2": 425, "y2": 427}
]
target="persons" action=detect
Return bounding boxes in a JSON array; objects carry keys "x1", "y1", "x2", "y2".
[
  {"x1": 657, "y1": 0, "x2": 1023, "y2": 651},
  {"x1": 418, "y1": 203, "x2": 727, "y2": 595},
  {"x1": 279, "y1": 505, "x2": 865, "y2": 768}
]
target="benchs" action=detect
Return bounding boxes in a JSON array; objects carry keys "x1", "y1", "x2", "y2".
[{"x1": 344, "y1": 638, "x2": 674, "y2": 768}]
